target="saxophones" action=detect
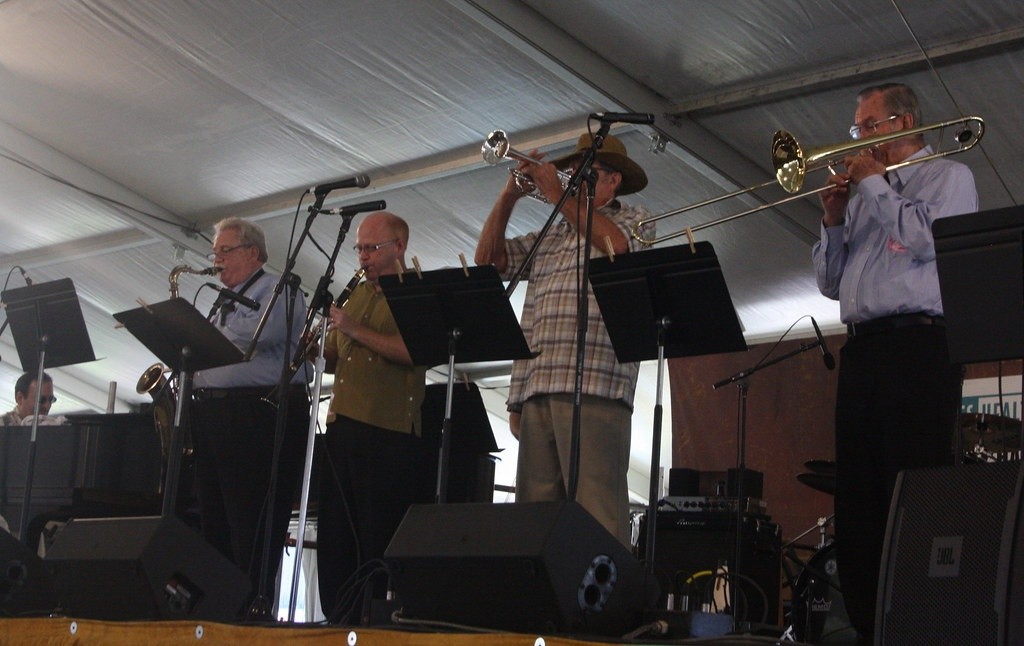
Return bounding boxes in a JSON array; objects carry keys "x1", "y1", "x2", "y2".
[{"x1": 135, "y1": 263, "x2": 224, "y2": 460}]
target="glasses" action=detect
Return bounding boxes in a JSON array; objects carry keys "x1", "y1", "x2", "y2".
[
  {"x1": 39, "y1": 394, "x2": 57, "y2": 403},
  {"x1": 206, "y1": 244, "x2": 251, "y2": 263},
  {"x1": 848, "y1": 116, "x2": 897, "y2": 140},
  {"x1": 352, "y1": 239, "x2": 397, "y2": 254},
  {"x1": 563, "y1": 166, "x2": 610, "y2": 175}
]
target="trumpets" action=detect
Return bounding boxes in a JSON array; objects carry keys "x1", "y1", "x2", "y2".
[{"x1": 478, "y1": 128, "x2": 582, "y2": 205}]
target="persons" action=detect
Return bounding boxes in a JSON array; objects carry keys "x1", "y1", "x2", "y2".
[
  {"x1": 298, "y1": 211, "x2": 426, "y2": 628},
  {"x1": 810, "y1": 82, "x2": 981, "y2": 644},
  {"x1": 175, "y1": 216, "x2": 314, "y2": 623},
  {"x1": 0, "y1": 371, "x2": 56, "y2": 427},
  {"x1": 475, "y1": 132, "x2": 657, "y2": 555}
]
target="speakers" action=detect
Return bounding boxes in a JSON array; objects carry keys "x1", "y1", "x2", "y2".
[
  {"x1": 638, "y1": 510, "x2": 782, "y2": 633},
  {"x1": 4, "y1": 514, "x2": 254, "y2": 624},
  {"x1": 874, "y1": 461, "x2": 1024, "y2": 646},
  {"x1": 384, "y1": 500, "x2": 654, "y2": 642}
]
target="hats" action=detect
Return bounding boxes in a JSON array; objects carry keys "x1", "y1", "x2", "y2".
[{"x1": 546, "y1": 131, "x2": 649, "y2": 197}]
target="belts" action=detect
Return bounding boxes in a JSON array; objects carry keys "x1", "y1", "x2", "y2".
[
  {"x1": 846, "y1": 312, "x2": 948, "y2": 335},
  {"x1": 194, "y1": 382, "x2": 307, "y2": 403}
]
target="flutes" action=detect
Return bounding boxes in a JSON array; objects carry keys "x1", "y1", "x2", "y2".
[{"x1": 260, "y1": 264, "x2": 367, "y2": 411}]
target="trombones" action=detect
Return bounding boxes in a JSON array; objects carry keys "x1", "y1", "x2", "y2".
[{"x1": 630, "y1": 113, "x2": 988, "y2": 247}]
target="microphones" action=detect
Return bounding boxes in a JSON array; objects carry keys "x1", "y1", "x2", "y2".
[
  {"x1": 307, "y1": 175, "x2": 371, "y2": 194},
  {"x1": 811, "y1": 316, "x2": 836, "y2": 371},
  {"x1": 321, "y1": 200, "x2": 386, "y2": 214},
  {"x1": 206, "y1": 283, "x2": 261, "y2": 311},
  {"x1": 19, "y1": 266, "x2": 34, "y2": 286},
  {"x1": 590, "y1": 111, "x2": 655, "y2": 125}
]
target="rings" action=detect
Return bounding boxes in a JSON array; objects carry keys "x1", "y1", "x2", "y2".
[{"x1": 332, "y1": 318, "x2": 333, "y2": 321}]
